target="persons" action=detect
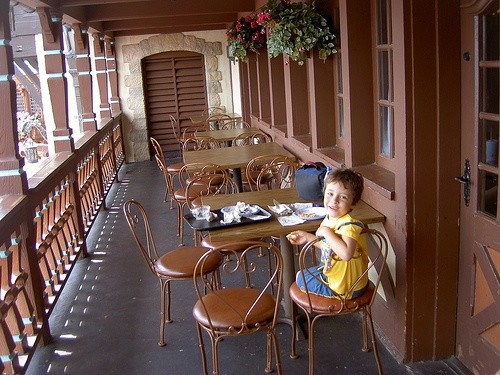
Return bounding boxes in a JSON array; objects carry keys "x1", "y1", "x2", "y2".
[{"x1": 287, "y1": 169, "x2": 369, "y2": 300}]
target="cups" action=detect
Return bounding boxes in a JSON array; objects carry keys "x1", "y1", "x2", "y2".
[{"x1": 189, "y1": 206, "x2": 210, "y2": 220}]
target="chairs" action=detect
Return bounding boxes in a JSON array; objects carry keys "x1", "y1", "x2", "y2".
[
  {"x1": 290, "y1": 229, "x2": 389, "y2": 375},
  {"x1": 123, "y1": 200, "x2": 224, "y2": 346},
  {"x1": 150, "y1": 107, "x2": 300, "y2": 262},
  {"x1": 192, "y1": 241, "x2": 284, "y2": 375}
]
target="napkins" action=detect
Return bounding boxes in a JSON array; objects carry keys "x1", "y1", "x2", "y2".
[
  {"x1": 190, "y1": 200, "x2": 270, "y2": 224},
  {"x1": 267, "y1": 198, "x2": 314, "y2": 227}
]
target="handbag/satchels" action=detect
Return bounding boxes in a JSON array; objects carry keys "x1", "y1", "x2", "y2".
[{"x1": 296, "y1": 161, "x2": 327, "y2": 201}]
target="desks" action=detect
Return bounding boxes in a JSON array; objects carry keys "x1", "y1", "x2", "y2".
[
  {"x1": 190, "y1": 113, "x2": 241, "y2": 130},
  {"x1": 183, "y1": 142, "x2": 296, "y2": 193},
  {"x1": 202, "y1": 187, "x2": 386, "y2": 342},
  {"x1": 193, "y1": 127, "x2": 263, "y2": 148}
]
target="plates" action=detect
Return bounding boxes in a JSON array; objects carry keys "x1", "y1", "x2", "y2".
[
  {"x1": 220, "y1": 205, "x2": 260, "y2": 216},
  {"x1": 293, "y1": 207, "x2": 327, "y2": 220}
]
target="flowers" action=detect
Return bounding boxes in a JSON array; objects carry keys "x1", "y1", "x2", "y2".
[
  {"x1": 16, "y1": 111, "x2": 48, "y2": 147},
  {"x1": 225, "y1": 0, "x2": 337, "y2": 67}
]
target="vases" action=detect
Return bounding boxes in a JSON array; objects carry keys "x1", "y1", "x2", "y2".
[{"x1": 26, "y1": 147, "x2": 38, "y2": 163}]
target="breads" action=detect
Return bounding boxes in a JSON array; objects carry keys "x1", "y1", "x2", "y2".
[{"x1": 286, "y1": 234, "x2": 296, "y2": 239}]
